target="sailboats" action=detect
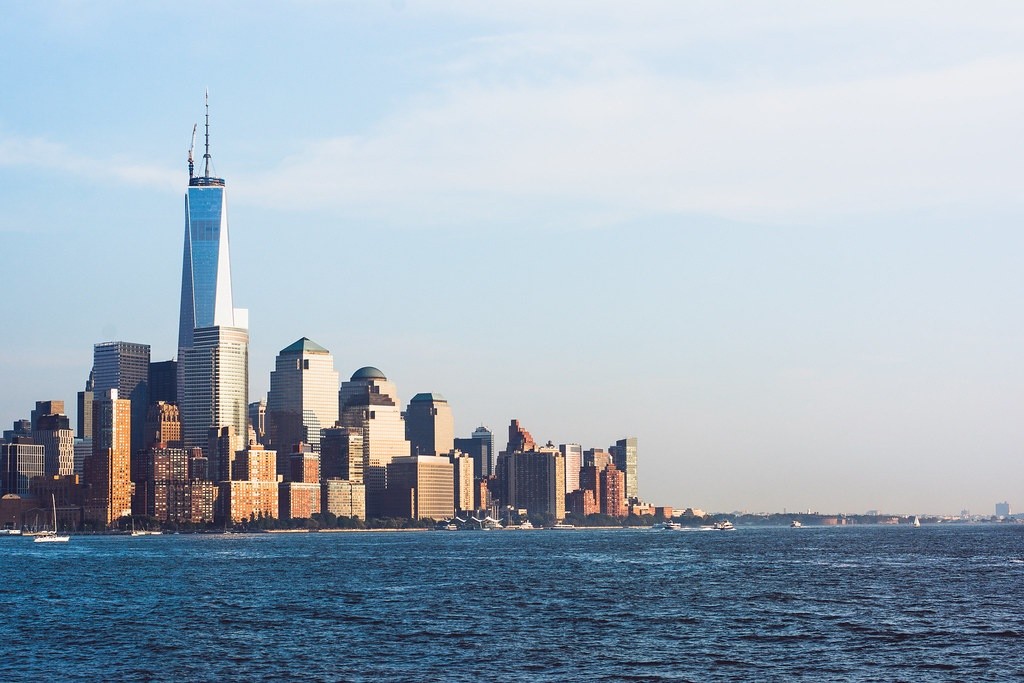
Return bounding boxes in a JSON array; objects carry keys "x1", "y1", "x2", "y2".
[
  {"x1": 131, "y1": 518, "x2": 139, "y2": 537},
  {"x1": 913, "y1": 515, "x2": 921, "y2": 528}
]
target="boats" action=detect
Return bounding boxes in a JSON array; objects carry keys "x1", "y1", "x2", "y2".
[
  {"x1": 23, "y1": 531, "x2": 56, "y2": 536},
  {"x1": 515, "y1": 523, "x2": 543, "y2": 529},
  {"x1": 448, "y1": 524, "x2": 458, "y2": 531},
  {"x1": 551, "y1": 525, "x2": 574, "y2": 530},
  {"x1": 653, "y1": 522, "x2": 682, "y2": 530},
  {"x1": 714, "y1": 519, "x2": 734, "y2": 530},
  {"x1": 790, "y1": 521, "x2": 801, "y2": 527},
  {"x1": 483, "y1": 520, "x2": 504, "y2": 531},
  {"x1": 33, "y1": 536, "x2": 71, "y2": 542}
]
